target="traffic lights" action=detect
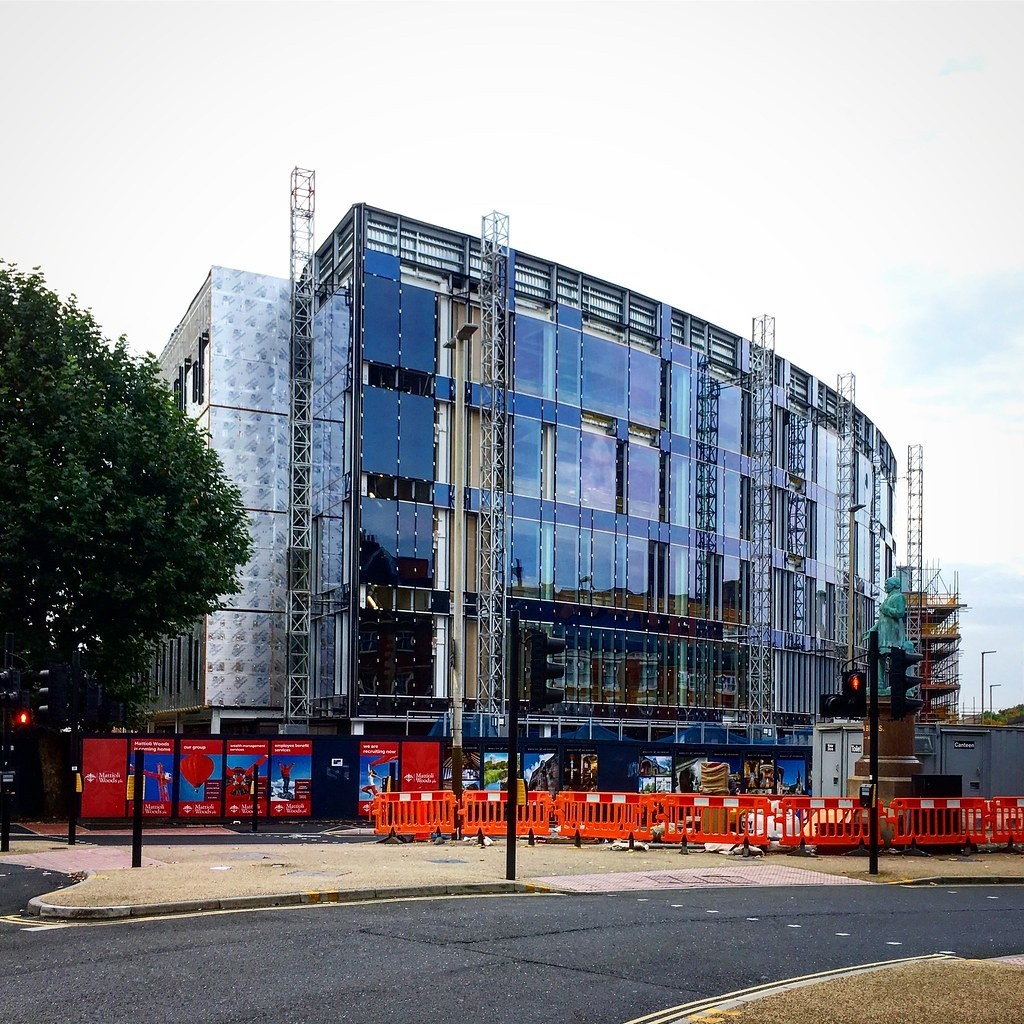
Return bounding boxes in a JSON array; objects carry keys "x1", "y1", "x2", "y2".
[
  {"x1": 36, "y1": 669, "x2": 51, "y2": 718},
  {"x1": 842, "y1": 671, "x2": 866, "y2": 718},
  {"x1": 14, "y1": 691, "x2": 32, "y2": 725},
  {"x1": 890, "y1": 646, "x2": 923, "y2": 718},
  {"x1": 819, "y1": 692, "x2": 844, "y2": 717},
  {"x1": 0, "y1": 666, "x2": 22, "y2": 711},
  {"x1": 526, "y1": 628, "x2": 566, "y2": 710}
]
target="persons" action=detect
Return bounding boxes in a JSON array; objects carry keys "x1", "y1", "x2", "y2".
[{"x1": 862, "y1": 577, "x2": 913, "y2": 696}]
